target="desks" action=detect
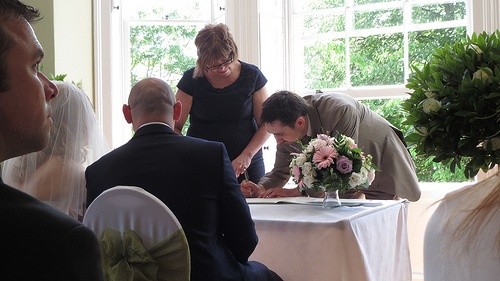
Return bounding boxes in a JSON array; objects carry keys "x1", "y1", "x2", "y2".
[{"x1": 246, "y1": 197, "x2": 412, "y2": 281}]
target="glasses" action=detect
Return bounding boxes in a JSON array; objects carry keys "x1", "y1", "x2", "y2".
[{"x1": 204, "y1": 55, "x2": 232, "y2": 70}]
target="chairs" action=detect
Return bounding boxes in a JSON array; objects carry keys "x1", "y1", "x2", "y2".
[{"x1": 81, "y1": 184, "x2": 192, "y2": 281}]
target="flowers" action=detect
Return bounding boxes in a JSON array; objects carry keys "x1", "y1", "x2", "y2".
[
  {"x1": 397, "y1": 29, "x2": 500, "y2": 178},
  {"x1": 290, "y1": 129, "x2": 375, "y2": 194}
]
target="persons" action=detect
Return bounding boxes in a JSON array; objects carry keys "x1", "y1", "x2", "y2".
[
  {"x1": 1, "y1": 80, "x2": 109, "y2": 223},
  {"x1": 240, "y1": 90, "x2": 421, "y2": 203},
  {"x1": 0, "y1": 0, "x2": 107, "y2": 281},
  {"x1": 85, "y1": 77, "x2": 283, "y2": 281},
  {"x1": 422, "y1": 170, "x2": 500, "y2": 281},
  {"x1": 174, "y1": 23, "x2": 271, "y2": 184}
]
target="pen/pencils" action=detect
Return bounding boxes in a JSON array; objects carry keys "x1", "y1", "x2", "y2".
[{"x1": 242, "y1": 165, "x2": 253, "y2": 198}]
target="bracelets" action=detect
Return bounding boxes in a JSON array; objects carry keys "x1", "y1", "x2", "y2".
[{"x1": 296, "y1": 185, "x2": 308, "y2": 197}]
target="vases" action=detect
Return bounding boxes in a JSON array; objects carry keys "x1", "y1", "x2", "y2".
[{"x1": 321, "y1": 189, "x2": 341, "y2": 209}]
